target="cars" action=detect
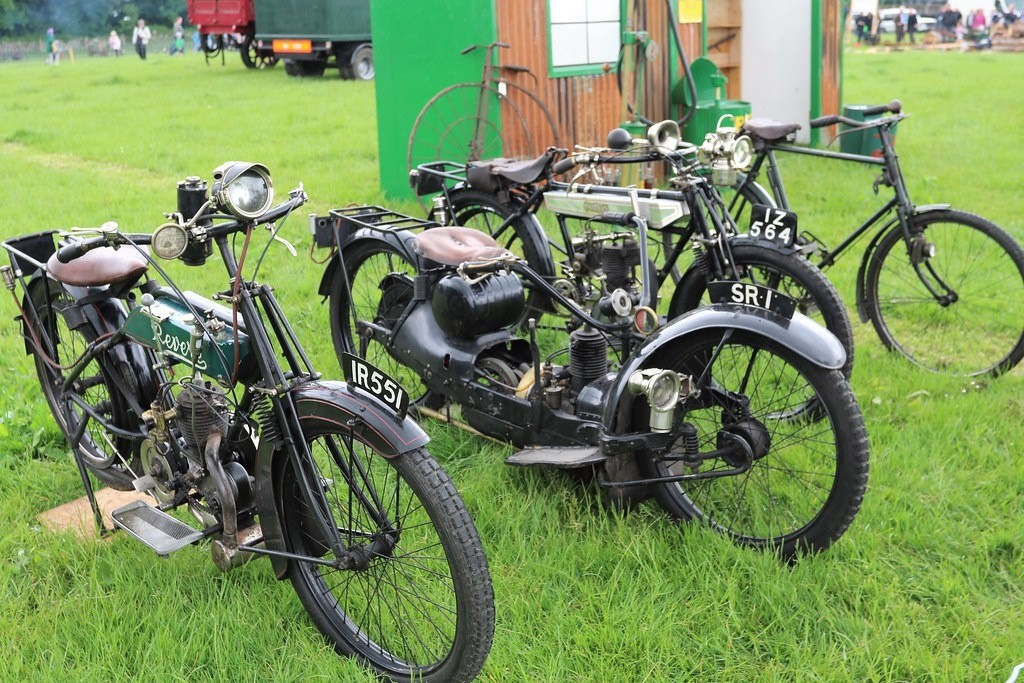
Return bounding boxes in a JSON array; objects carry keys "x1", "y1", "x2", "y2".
[{"x1": 879, "y1": 8, "x2": 936, "y2": 35}]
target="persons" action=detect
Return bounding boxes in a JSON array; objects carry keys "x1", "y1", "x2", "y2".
[
  {"x1": 110, "y1": 31, "x2": 120, "y2": 56},
  {"x1": 986, "y1": 4, "x2": 1018, "y2": 40},
  {"x1": 45, "y1": 28, "x2": 60, "y2": 66},
  {"x1": 855, "y1": 12, "x2": 873, "y2": 44},
  {"x1": 169, "y1": 17, "x2": 184, "y2": 55},
  {"x1": 967, "y1": 9, "x2": 986, "y2": 39},
  {"x1": 934, "y1": 5, "x2": 962, "y2": 42},
  {"x1": 132, "y1": 19, "x2": 151, "y2": 58},
  {"x1": 895, "y1": 5, "x2": 917, "y2": 42},
  {"x1": 192, "y1": 32, "x2": 243, "y2": 52}
]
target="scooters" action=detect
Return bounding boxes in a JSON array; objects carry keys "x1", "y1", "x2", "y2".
[
  {"x1": 0, "y1": 160, "x2": 497, "y2": 683},
  {"x1": 308, "y1": 115, "x2": 871, "y2": 564}
]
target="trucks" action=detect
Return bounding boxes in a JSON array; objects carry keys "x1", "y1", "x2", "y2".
[
  {"x1": 188, "y1": 0, "x2": 282, "y2": 71},
  {"x1": 254, "y1": 0, "x2": 377, "y2": 83}
]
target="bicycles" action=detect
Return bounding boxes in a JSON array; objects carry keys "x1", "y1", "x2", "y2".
[{"x1": 662, "y1": 99, "x2": 1024, "y2": 382}]
